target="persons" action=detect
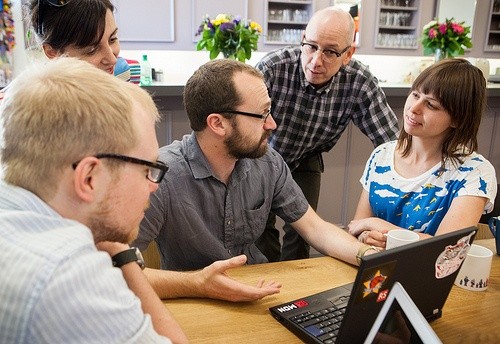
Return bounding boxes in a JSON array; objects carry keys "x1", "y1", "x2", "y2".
[
  {"x1": 0, "y1": 55, "x2": 189, "y2": 344},
  {"x1": 28, "y1": 0, "x2": 122, "y2": 75},
  {"x1": 347, "y1": 58, "x2": 497, "y2": 251},
  {"x1": 253, "y1": 6, "x2": 400, "y2": 263},
  {"x1": 128, "y1": 59, "x2": 380, "y2": 302}
]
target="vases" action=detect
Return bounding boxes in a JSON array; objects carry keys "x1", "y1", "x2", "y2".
[{"x1": 431, "y1": 49, "x2": 444, "y2": 63}]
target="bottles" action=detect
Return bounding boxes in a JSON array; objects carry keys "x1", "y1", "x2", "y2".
[{"x1": 140, "y1": 55, "x2": 152, "y2": 86}]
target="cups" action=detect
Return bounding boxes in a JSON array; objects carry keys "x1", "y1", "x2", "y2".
[
  {"x1": 454, "y1": 244, "x2": 493, "y2": 291},
  {"x1": 489, "y1": 216, "x2": 500, "y2": 256},
  {"x1": 269, "y1": 8, "x2": 308, "y2": 22},
  {"x1": 378, "y1": 0, "x2": 417, "y2": 46},
  {"x1": 269, "y1": 28, "x2": 305, "y2": 43},
  {"x1": 382, "y1": 229, "x2": 420, "y2": 250}
]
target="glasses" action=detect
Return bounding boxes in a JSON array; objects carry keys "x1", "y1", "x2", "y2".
[
  {"x1": 205, "y1": 107, "x2": 272, "y2": 123},
  {"x1": 300, "y1": 35, "x2": 352, "y2": 63},
  {"x1": 72, "y1": 153, "x2": 168, "y2": 183}
]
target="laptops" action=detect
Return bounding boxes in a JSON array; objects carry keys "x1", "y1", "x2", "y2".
[{"x1": 269, "y1": 227, "x2": 479, "y2": 344}]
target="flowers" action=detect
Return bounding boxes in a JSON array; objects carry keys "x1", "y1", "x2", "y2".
[
  {"x1": 420, "y1": 17, "x2": 473, "y2": 62},
  {"x1": 195, "y1": 13, "x2": 262, "y2": 62}
]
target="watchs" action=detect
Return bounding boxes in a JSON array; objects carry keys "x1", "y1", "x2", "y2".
[{"x1": 113, "y1": 247, "x2": 145, "y2": 271}]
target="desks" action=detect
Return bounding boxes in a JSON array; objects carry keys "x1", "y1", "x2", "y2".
[{"x1": 159, "y1": 238, "x2": 500, "y2": 344}]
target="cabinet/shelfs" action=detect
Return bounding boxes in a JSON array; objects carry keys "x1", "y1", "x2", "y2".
[
  {"x1": 247, "y1": 0, "x2": 500, "y2": 56},
  {"x1": 148, "y1": 95, "x2": 500, "y2": 257}
]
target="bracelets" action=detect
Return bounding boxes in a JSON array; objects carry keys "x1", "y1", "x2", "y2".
[{"x1": 356, "y1": 245, "x2": 380, "y2": 265}]
